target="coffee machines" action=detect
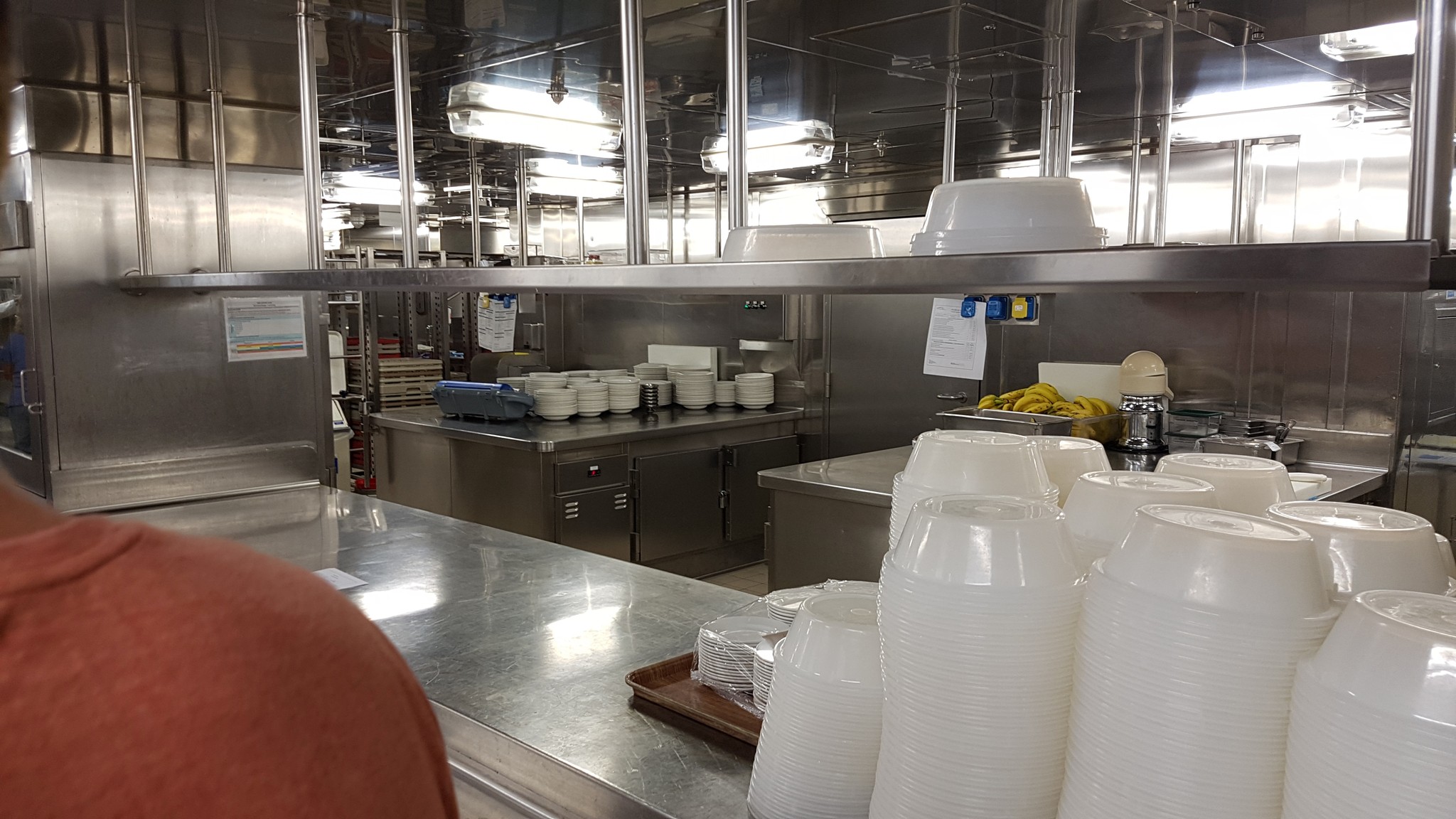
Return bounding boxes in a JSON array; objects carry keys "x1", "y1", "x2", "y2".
[{"x1": 1114, "y1": 351, "x2": 1174, "y2": 454}]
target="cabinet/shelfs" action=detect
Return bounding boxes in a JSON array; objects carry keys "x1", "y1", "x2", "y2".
[{"x1": 628, "y1": 435, "x2": 802, "y2": 578}]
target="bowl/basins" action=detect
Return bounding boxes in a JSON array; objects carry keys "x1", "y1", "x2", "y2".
[
  {"x1": 743, "y1": 429, "x2": 1454, "y2": 818},
  {"x1": 909, "y1": 177, "x2": 1109, "y2": 257},
  {"x1": 715, "y1": 226, "x2": 882, "y2": 262}
]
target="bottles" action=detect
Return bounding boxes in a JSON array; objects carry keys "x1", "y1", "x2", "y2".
[{"x1": 1118, "y1": 396, "x2": 1163, "y2": 449}]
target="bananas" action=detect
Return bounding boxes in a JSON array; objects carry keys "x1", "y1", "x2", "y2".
[{"x1": 978, "y1": 382, "x2": 1118, "y2": 442}]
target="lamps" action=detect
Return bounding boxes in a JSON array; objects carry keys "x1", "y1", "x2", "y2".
[
  {"x1": 701, "y1": 119, "x2": 835, "y2": 153},
  {"x1": 1157, "y1": 100, "x2": 1368, "y2": 146},
  {"x1": 1172, "y1": 79, "x2": 1366, "y2": 118},
  {"x1": 446, "y1": 105, "x2": 622, "y2": 152},
  {"x1": 700, "y1": 140, "x2": 835, "y2": 175},
  {"x1": 446, "y1": 81, "x2": 623, "y2": 128}
]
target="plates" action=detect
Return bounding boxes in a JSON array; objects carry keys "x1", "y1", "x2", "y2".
[
  {"x1": 697, "y1": 579, "x2": 878, "y2": 716},
  {"x1": 495, "y1": 362, "x2": 774, "y2": 421}
]
